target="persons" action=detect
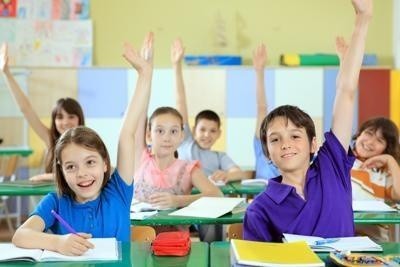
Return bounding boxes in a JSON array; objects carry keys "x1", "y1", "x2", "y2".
[
  {"x1": 170, "y1": 36, "x2": 242, "y2": 240},
  {"x1": 1, "y1": 38, "x2": 85, "y2": 184},
  {"x1": 11, "y1": 39, "x2": 153, "y2": 256},
  {"x1": 249, "y1": 40, "x2": 282, "y2": 180},
  {"x1": 242, "y1": 0, "x2": 375, "y2": 243},
  {"x1": 334, "y1": 34, "x2": 400, "y2": 241},
  {"x1": 133, "y1": 30, "x2": 224, "y2": 240}
]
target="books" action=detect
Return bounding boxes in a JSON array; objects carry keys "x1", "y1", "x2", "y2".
[
  {"x1": 242, "y1": 177, "x2": 268, "y2": 186},
  {"x1": 169, "y1": 196, "x2": 247, "y2": 218},
  {"x1": 129, "y1": 196, "x2": 160, "y2": 212},
  {"x1": 1, "y1": 237, "x2": 123, "y2": 262},
  {"x1": 352, "y1": 198, "x2": 398, "y2": 211},
  {"x1": 282, "y1": 231, "x2": 383, "y2": 252},
  {"x1": 1, "y1": 177, "x2": 57, "y2": 187},
  {"x1": 231, "y1": 237, "x2": 325, "y2": 266}
]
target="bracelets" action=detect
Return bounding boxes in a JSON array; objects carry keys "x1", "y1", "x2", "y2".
[{"x1": 177, "y1": 194, "x2": 185, "y2": 209}]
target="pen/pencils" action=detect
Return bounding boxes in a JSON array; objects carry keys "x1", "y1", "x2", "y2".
[
  {"x1": 51, "y1": 210, "x2": 81, "y2": 236},
  {"x1": 315, "y1": 240, "x2": 339, "y2": 245}
]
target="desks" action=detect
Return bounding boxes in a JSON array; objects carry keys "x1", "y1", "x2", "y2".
[{"x1": 0, "y1": 148, "x2": 34, "y2": 182}]
